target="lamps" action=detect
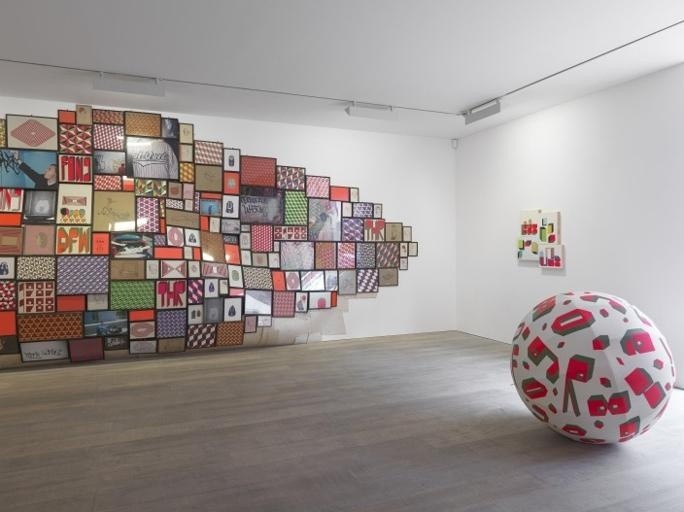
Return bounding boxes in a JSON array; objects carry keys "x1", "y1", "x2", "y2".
[
  {"x1": 345, "y1": 99, "x2": 393, "y2": 119},
  {"x1": 465, "y1": 99, "x2": 500, "y2": 125},
  {"x1": 91, "y1": 72, "x2": 165, "y2": 96}
]
